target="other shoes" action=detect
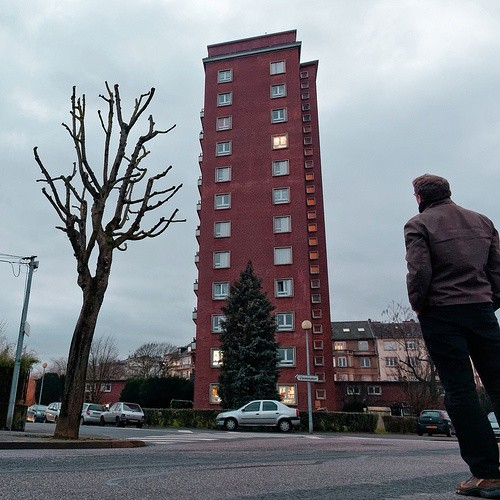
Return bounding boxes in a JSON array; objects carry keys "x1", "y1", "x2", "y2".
[{"x1": 454, "y1": 475, "x2": 500, "y2": 499}]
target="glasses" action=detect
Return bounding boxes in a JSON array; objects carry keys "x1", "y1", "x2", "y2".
[{"x1": 414, "y1": 193, "x2": 416, "y2": 196}]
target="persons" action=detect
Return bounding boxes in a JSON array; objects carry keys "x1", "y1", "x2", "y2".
[{"x1": 404, "y1": 174, "x2": 500, "y2": 500}]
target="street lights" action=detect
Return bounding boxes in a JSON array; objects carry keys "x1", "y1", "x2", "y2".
[
  {"x1": 302, "y1": 320, "x2": 314, "y2": 435},
  {"x1": 38, "y1": 362, "x2": 48, "y2": 405}
]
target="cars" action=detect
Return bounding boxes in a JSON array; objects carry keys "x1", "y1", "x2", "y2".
[
  {"x1": 215, "y1": 400, "x2": 301, "y2": 433},
  {"x1": 487, "y1": 412, "x2": 500, "y2": 438}
]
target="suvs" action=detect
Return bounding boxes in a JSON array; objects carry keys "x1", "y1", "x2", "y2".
[
  {"x1": 100, "y1": 402, "x2": 144, "y2": 429},
  {"x1": 80, "y1": 403, "x2": 105, "y2": 426},
  {"x1": 26, "y1": 402, "x2": 62, "y2": 424},
  {"x1": 419, "y1": 409, "x2": 456, "y2": 437}
]
